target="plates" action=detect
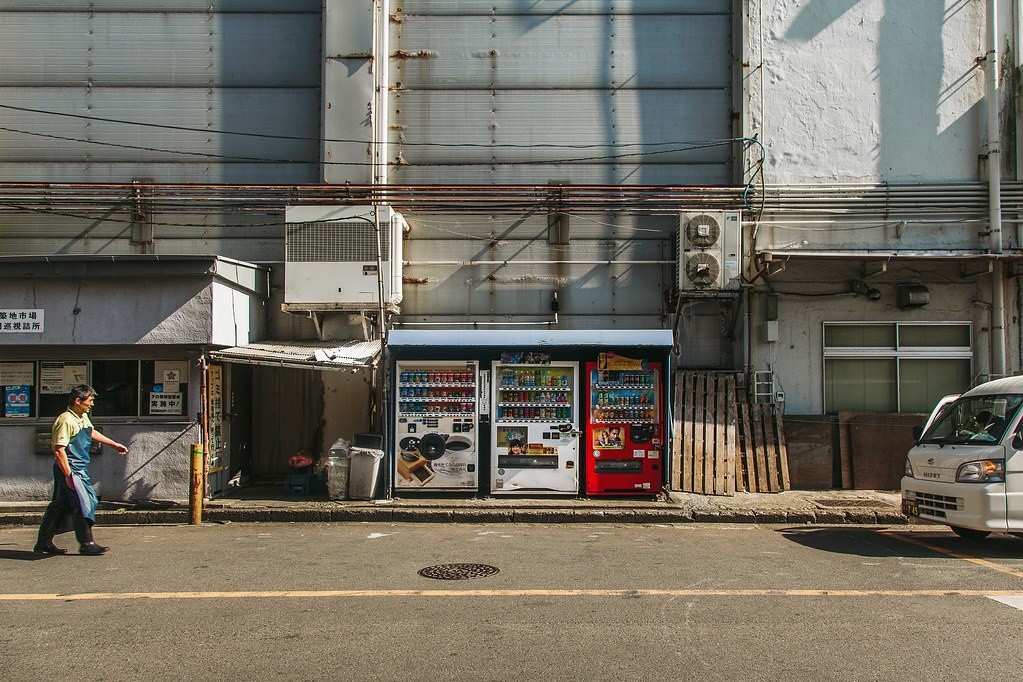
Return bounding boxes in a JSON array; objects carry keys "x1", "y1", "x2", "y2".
[{"x1": 431, "y1": 452, "x2": 476, "y2": 478}]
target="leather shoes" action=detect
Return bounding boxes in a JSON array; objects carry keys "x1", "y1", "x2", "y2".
[
  {"x1": 34, "y1": 543, "x2": 67, "y2": 554},
  {"x1": 79, "y1": 541, "x2": 109, "y2": 554}
]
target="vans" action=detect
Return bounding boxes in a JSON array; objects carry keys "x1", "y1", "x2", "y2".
[{"x1": 900, "y1": 376, "x2": 1023, "y2": 536}]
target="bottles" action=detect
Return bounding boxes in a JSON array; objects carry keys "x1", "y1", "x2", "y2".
[
  {"x1": 502, "y1": 370, "x2": 568, "y2": 418},
  {"x1": 594, "y1": 373, "x2": 655, "y2": 419}
]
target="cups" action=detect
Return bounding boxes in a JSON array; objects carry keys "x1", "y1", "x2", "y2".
[{"x1": 401, "y1": 372, "x2": 474, "y2": 412}]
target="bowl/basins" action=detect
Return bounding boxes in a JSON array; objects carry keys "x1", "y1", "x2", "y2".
[{"x1": 445, "y1": 436, "x2": 473, "y2": 459}]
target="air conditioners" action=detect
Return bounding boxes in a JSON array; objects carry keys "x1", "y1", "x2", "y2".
[
  {"x1": 674, "y1": 209, "x2": 741, "y2": 292},
  {"x1": 282, "y1": 203, "x2": 396, "y2": 305}
]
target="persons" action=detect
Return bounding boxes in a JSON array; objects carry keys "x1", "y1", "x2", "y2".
[
  {"x1": 509, "y1": 439, "x2": 524, "y2": 455},
  {"x1": 599, "y1": 427, "x2": 621, "y2": 446},
  {"x1": 976, "y1": 411, "x2": 1023, "y2": 449},
  {"x1": 34, "y1": 385, "x2": 128, "y2": 557}
]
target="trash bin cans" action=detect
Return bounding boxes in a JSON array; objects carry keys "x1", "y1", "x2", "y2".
[
  {"x1": 348, "y1": 446, "x2": 384, "y2": 500},
  {"x1": 327, "y1": 441, "x2": 350, "y2": 500}
]
